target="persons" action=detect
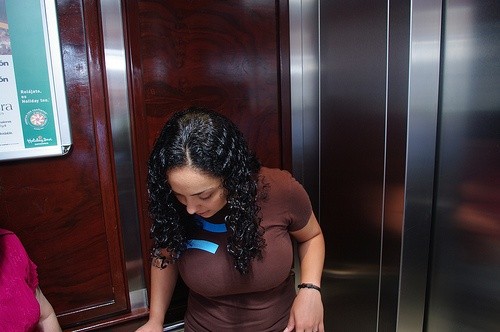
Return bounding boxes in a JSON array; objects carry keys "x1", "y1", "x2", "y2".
[
  {"x1": 0, "y1": 229, "x2": 63, "y2": 332},
  {"x1": 134, "y1": 107, "x2": 325, "y2": 332}
]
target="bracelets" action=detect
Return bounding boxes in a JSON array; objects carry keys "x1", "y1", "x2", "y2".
[{"x1": 298, "y1": 283, "x2": 321, "y2": 293}]
